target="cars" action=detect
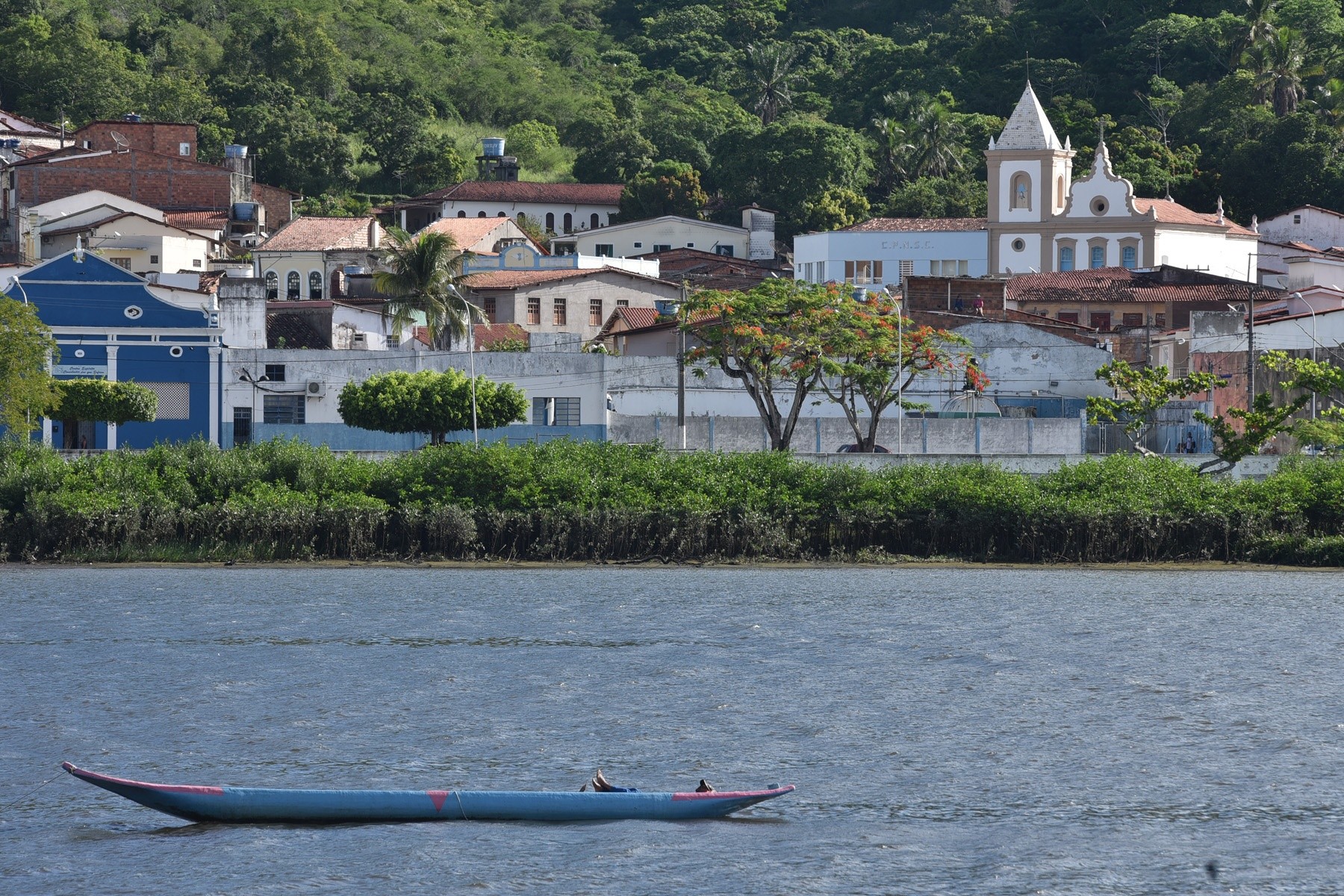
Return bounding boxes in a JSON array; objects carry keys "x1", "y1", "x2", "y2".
[{"x1": 837, "y1": 444, "x2": 891, "y2": 454}]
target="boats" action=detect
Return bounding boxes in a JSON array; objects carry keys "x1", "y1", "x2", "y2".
[{"x1": 59, "y1": 761, "x2": 796, "y2": 825}]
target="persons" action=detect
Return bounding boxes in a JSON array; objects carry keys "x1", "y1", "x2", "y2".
[
  {"x1": 955, "y1": 294, "x2": 964, "y2": 314},
  {"x1": 1182, "y1": 432, "x2": 1196, "y2": 453},
  {"x1": 973, "y1": 294, "x2": 984, "y2": 317},
  {"x1": 592, "y1": 769, "x2": 713, "y2": 794}
]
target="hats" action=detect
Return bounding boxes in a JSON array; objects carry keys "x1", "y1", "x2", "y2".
[{"x1": 700, "y1": 779, "x2": 715, "y2": 793}]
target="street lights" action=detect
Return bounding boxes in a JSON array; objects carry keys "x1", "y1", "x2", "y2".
[
  {"x1": 1293, "y1": 291, "x2": 1316, "y2": 421},
  {"x1": 447, "y1": 284, "x2": 478, "y2": 449},
  {"x1": 238, "y1": 373, "x2": 270, "y2": 444},
  {"x1": 882, "y1": 287, "x2": 901, "y2": 454}
]
